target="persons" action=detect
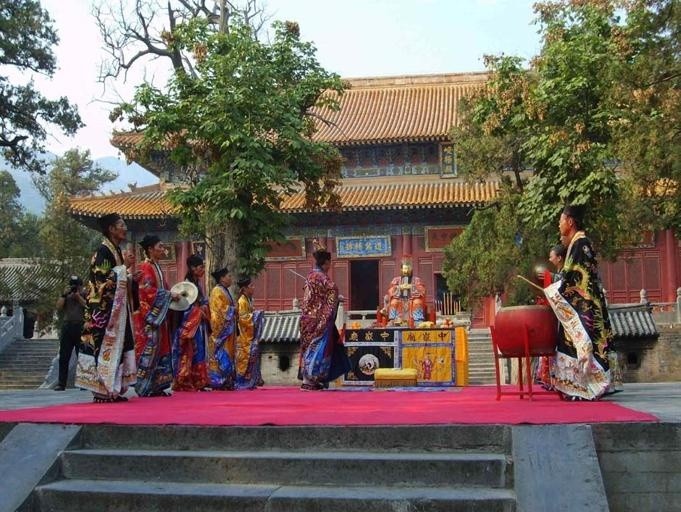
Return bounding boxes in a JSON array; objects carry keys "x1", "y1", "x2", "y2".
[
  {"x1": 379, "y1": 253, "x2": 428, "y2": 330},
  {"x1": 297, "y1": 248, "x2": 351, "y2": 391},
  {"x1": 534, "y1": 206, "x2": 624, "y2": 402}
]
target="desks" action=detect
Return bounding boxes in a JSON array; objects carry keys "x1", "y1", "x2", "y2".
[{"x1": 338, "y1": 321, "x2": 471, "y2": 389}]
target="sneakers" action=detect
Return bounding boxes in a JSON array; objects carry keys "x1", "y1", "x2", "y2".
[
  {"x1": 201, "y1": 384, "x2": 225, "y2": 392},
  {"x1": 299, "y1": 383, "x2": 323, "y2": 392},
  {"x1": 118, "y1": 389, "x2": 137, "y2": 400},
  {"x1": 143, "y1": 391, "x2": 171, "y2": 397},
  {"x1": 92, "y1": 394, "x2": 127, "y2": 403}
]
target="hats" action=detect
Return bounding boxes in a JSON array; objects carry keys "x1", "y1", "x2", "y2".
[
  {"x1": 312, "y1": 251, "x2": 331, "y2": 262},
  {"x1": 563, "y1": 205, "x2": 580, "y2": 218},
  {"x1": 138, "y1": 233, "x2": 160, "y2": 249},
  {"x1": 98, "y1": 212, "x2": 120, "y2": 227},
  {"x1": 186, "y1": 254, "x2": 202, "y2": 266},
  {"x1": 210, "y1": 267, "x2": 228, "y2": 279},
  {"x1": 70, "y1": 275, "x2": 83, "y2": 284},
  {"x1": 235, "y1": 276, "x2": 250, "y2": 287}
]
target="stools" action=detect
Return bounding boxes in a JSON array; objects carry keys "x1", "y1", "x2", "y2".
[{"x1": 370, "y1": 366, "x2": 419, "y2": 388}]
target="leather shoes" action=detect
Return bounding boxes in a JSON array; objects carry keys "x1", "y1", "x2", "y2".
[{"x1": 53, "y1": 386, "x2": 65, "y2": 391}]
